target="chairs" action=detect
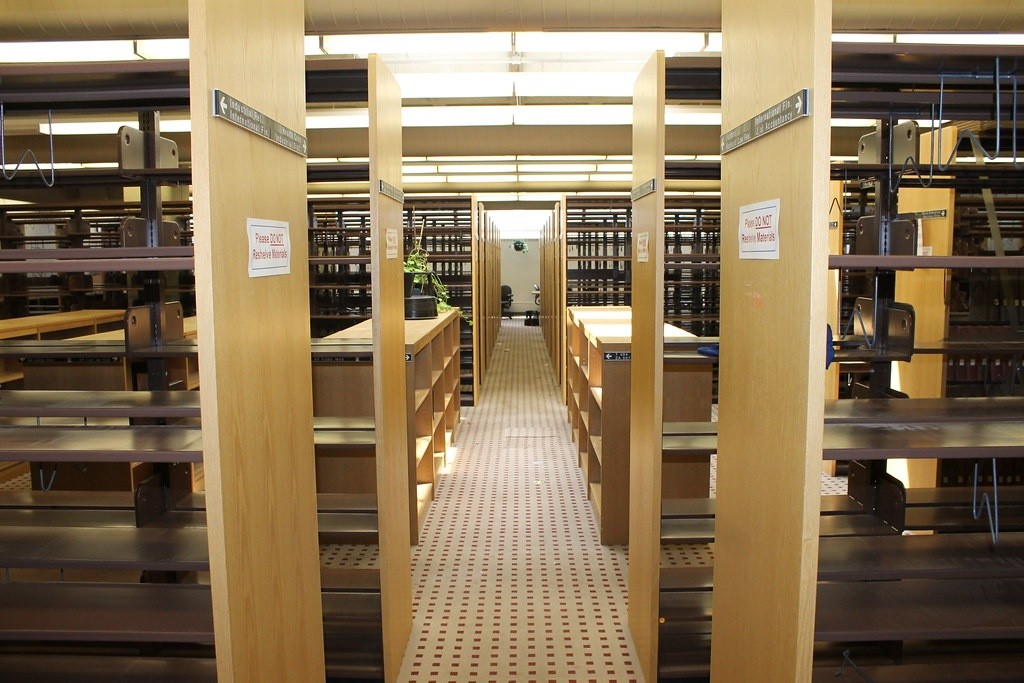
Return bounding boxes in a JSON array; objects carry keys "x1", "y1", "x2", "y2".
[
  {"x1": 501, "y1": 284, "x2": 514, "y2": 319},
  {"x1": 534, "y1": 283, "x2": 540, "y2": 305}
]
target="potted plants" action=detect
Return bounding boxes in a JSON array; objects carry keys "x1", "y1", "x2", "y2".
[
  {"x1": 508, "y1": 239, "x2": 528, "y2": 253},
  {"x1": 403, "y1": 246, "x2": 473, "y2": 326}
]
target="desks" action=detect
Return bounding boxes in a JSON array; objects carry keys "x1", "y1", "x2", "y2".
[{"x1": 531, "y1": 291, "x2": 540, "y2": 295}]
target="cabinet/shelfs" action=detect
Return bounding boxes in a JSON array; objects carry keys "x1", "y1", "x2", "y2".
[{"x1": 0, "y1": 0, "x2": 1024, "y2": 683}]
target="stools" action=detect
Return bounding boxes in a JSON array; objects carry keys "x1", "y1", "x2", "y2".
[
  {"x1": 523, "y1": 310, "x2": 539, "y2": 325},
  {"x1": 403, "y1": 271, "x2": 438, "y2": 319}
]
